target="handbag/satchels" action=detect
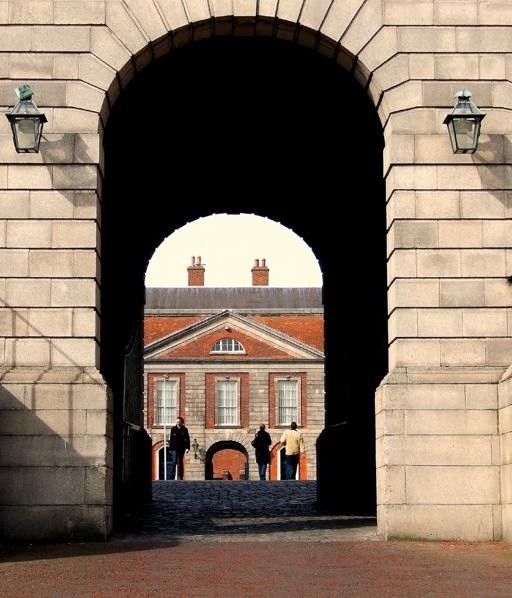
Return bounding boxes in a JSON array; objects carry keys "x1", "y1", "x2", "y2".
[{"x1": 251, "y1": 437, "x2": 259, "y2": 448}]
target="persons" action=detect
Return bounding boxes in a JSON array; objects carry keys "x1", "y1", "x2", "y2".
[
  {"x1": 169, "y1": 417, "x2": 190, "y2": 480},
  {"x1": 280, "y1": 422, "x2": 306, "y2": 480},
  {"x1": 251, "y1": 423, "x2": 271, "y2": 480}
]
